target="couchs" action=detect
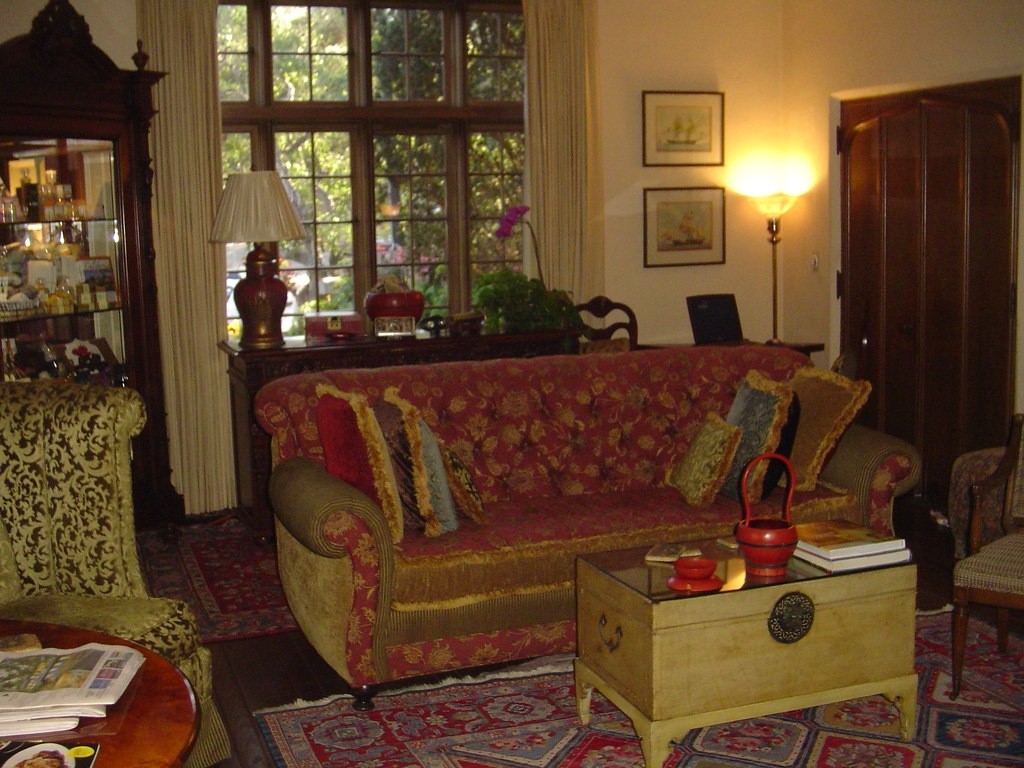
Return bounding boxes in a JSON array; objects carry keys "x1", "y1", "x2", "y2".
[{"x1": 254, "y1": 345, "x2": 923, "y2": 712}]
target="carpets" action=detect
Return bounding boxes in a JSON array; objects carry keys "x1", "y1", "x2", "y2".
[
  {"x1": 137, "y1": 513, "x2": 299, "y2": 642},
  {"x1": 255, "y1": 605, "x2": 1024, "y2": 768}
]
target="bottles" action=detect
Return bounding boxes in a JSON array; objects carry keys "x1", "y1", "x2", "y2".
[{"x1": 0, "y1": 165, "x2": 83, "y2": 318}]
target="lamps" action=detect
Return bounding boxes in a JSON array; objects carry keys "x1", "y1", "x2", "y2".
[
  {"x1": 752, "y1": 195, "x2": 795, "y2": 345},
  {"x1": 207, "y1": 171, "x2": 305, "y2": 353}
]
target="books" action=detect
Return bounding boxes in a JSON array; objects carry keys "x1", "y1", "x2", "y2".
[{"x1": 792, "y1": 518, "x2": 912, "y2": 573}]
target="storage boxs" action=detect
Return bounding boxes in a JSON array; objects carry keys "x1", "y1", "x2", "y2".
[{"x1": 306, "y1": 312, "x2": 365, "y2": 344}]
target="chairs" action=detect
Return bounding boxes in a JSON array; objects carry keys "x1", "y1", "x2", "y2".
[
  {"x1": 949, "y1": 414, "x2": 1024, "y2": 702},
  {"x1": 0, "y1": 382, "x2": 232, "y2": 768},
  {"x1": 569, "y1": 296, "x2": 638, "y2": 345}
]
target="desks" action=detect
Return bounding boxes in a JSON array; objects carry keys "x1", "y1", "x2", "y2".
[{"x1": 1, "y1": 620, "x2": 202, "y2": 768}]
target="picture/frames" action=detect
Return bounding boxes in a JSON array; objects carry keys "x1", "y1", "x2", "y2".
[
  {"x1": 643, "y1": 187, "x2": 725, "y2": 268},
  {"x1": 641, "y1": 91, "x2": 726, "y2": 165}
]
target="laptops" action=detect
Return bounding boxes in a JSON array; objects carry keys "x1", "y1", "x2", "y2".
[{"x1": 687, "y1": 293, "x2": 743, "y2": 345}]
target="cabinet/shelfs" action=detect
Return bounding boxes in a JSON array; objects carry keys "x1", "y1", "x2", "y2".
[
  {"x1": 216, "y1": 328, "x2": 569, "y2": 544},
  {"x1": 1, "y1": 1, "x2": 187, "y2": 542}
]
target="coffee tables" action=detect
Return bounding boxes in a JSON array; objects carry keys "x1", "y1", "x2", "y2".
[{"x1": 574, "y1": 536, "x2": 916, "y2": 768}]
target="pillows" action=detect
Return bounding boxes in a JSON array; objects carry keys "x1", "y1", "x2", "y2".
[
  {"x1": 309, "y1": 382, "x2": 488, "y2": 544},
  {"x1": 666, "y1": 353, "x2": 869, "y2": 504}
]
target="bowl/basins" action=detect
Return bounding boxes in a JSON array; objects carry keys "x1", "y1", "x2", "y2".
[
  {"x1": 366, "y1": 290, "x2": 424, "y2": 324},
  {"x1": 675, "y1": 557, "x2": 718, "y2": 579}
]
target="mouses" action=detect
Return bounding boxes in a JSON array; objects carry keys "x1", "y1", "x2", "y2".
[{"x1": 766, "y1": 339, "x2": 782, "y2": 347}]
